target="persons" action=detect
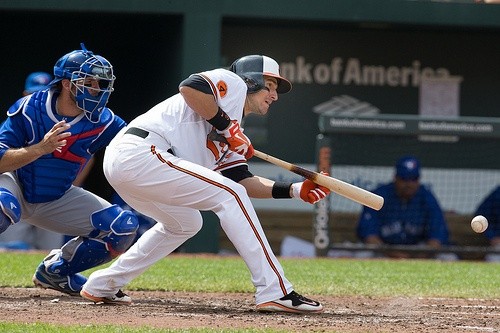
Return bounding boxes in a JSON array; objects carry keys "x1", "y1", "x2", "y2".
[
  {"x1": 0, "y1": 43, "x2": 140, "y2": 296},
  {"x1": 354, "y1": 155, "x2": 500, "y2": 261},
  {"x1": 78, "y1": 55, "x2": 329, "y2": 315},
  {"x1": 23, "y1": 72, "x2": 94, "y2": 187}
]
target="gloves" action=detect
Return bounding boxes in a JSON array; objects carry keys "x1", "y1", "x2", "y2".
[
  {"x1": 292, "y1": 171, "x2": 330, "y2": 204},
  {"x1": 216, "y1": 119, "x2": 254, "y2": 160}
]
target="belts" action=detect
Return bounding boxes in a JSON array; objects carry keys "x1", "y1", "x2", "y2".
[{"x1": 125, "y1": 127, "x2": 174, "y2": 155}]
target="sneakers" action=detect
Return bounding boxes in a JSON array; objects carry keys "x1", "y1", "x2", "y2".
[
  {"x1": 33, "y1": 262, "x2": 89, "y2": 296},
  {"x1": 256, "y1": 290, "x2": 323, "y2": 313},
  {"x1": 80, "y1": 288, "x2": 131, "y2": 305}
]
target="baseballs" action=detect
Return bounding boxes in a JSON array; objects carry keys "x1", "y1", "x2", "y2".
[{"x1": 471, "y1": 216, "x2": 488, "y2": 233}]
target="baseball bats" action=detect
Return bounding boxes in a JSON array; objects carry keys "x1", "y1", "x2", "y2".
[{"x1": 208, "y1": 129, "x2": 385, "y2": 212}]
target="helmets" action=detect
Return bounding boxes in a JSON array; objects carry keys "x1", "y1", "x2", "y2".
[
  {"x1": 48, "y1": 50, "x2": 110, "y2": 85},
  {"x1": 232, "y1": 54, "x2": 291, "y2": 94}
]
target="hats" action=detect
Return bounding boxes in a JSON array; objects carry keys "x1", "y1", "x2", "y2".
[
  {"x1": 396, "y1": 157, "x2": 419, "y2": 180},
  {"x1": 25, "y1": 72, "x2": 52, "y2": 93}
]
